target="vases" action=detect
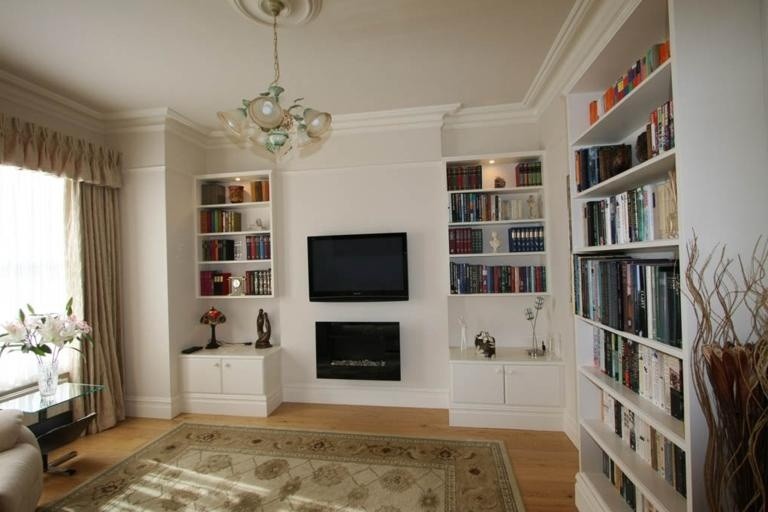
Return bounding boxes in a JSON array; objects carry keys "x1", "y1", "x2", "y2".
[
  {"x1": 36, "y1": 364, "x2": 58, "y2": 398},
  {"x1": 474, "y1": 329, "x2": 497, "y2": 358}
]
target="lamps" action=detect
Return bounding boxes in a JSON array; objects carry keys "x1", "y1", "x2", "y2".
[
  {"x1": 200, "y1": 305, "x2": 226, "y2": 351},
  {"x1": 216, "y1": 1, "x2": 333, "y2": 169}
]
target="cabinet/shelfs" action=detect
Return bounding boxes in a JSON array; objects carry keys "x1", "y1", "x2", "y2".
[
  {"x1": 193, "y1": 169, "x2": 279, "y2": 301},
  {"x1": 440, "y1": 148, "x2": 552, "y2": 296},
  {"x1": 178, "y1": 345, "x2": 282, "y2": 419},
  {"x1": 562, "y1": 3, "x2": 767, "y2": 512},
  {"x1": 443, "y1": 347, "x2": 566, "y2": 433}
]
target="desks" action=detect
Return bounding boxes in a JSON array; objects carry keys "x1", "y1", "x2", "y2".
[{"x1": 0, "y1": 378, "x2": 104, "y2": 477}]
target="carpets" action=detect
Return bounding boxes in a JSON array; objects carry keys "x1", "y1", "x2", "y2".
[{"x1": 37, "y1": 421, "x2": 528, "y2": 512}]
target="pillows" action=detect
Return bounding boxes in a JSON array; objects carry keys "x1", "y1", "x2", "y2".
[{"x1": 0, "y1": 408, "x2": 23, "y2": 454}]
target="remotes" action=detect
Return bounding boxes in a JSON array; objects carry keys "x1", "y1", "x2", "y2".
[{"x1": 183, "y1": 347, "x2": 202, "y2": 353}]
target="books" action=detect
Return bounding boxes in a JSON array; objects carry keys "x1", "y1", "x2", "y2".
[
  {"x1": 602, "y1": 450, "x2": 658, "y2": 512},
  {"x1": 589, "y1": 43, "x2": 670, "y2": 125},
  {"x1": 200, "y1": 180, "x2": 271, "y2": 296},
  {"x1": 592, "y1": 325, "x2": 685, "y2": 422},
  {"x1": 583, "y1": 179, "x2": 678, "y2": 246},
  {"x1": 573, "y1": 144, "x2": 631, "y2": 193},
  {"x1": 646, "y1": 99, "x2": 676, "y2": 161},
  {"x1": 600, "y1": 390, "x2": 687, "y2": 498},
  {"x1": 571, "y1": 254, "x2": 683, "y2": 349}
]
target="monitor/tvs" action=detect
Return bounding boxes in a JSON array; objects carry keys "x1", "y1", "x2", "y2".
[{"x1": 308, "y1": 232, "x2": 408, "y2": 302}]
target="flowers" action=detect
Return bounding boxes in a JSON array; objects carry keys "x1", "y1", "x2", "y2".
[{"x1": 1, "y1": 295, "x2": 99, "y2": 371}]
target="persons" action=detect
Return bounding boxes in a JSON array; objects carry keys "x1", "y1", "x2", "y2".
[
  {"x1": 257, "y1": 309, "x2": 271, "y2": 343},
  {"x1": 447, "y1": 161, "x2": 547, "y2": 294}
]
